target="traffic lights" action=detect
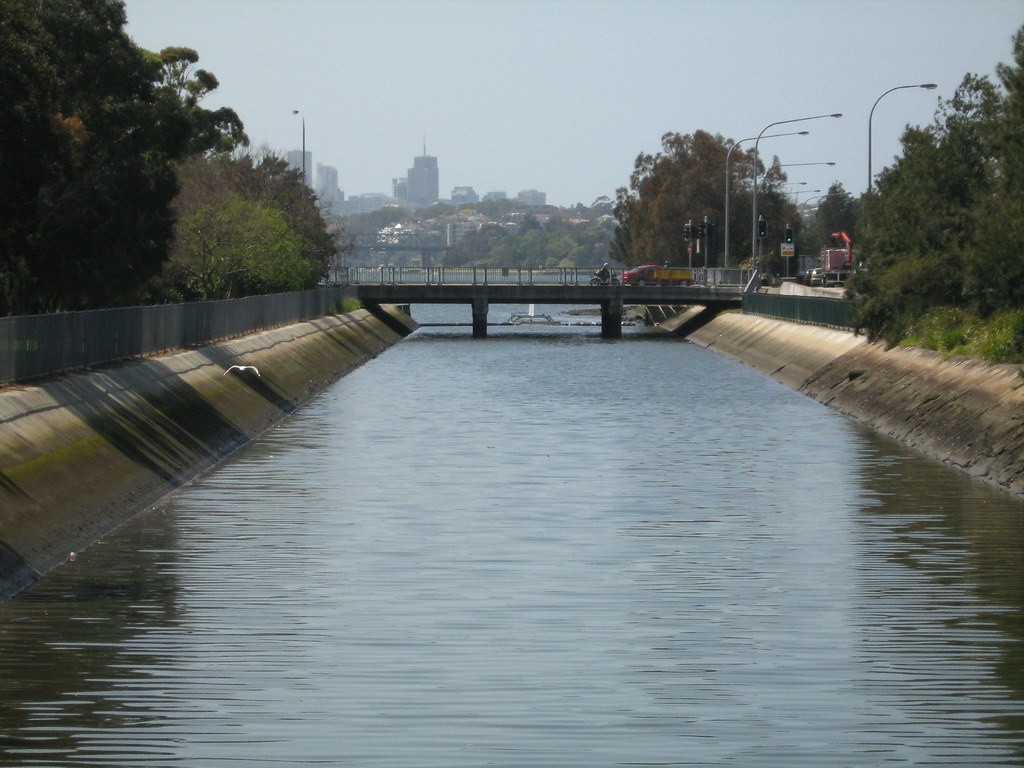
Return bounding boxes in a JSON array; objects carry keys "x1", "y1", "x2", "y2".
[
  {"x1": 708, "y1": 222, "x2": 716, "y2": 237},
  {"x1": 683, "y1": 224, "x2": 690, "y2": 242},
  {"x1": 698, "y1": 222, "x2": 704, "y2": 238},
  {"x1": 785, "y1": 229, "x2": 793, "y2": 244},
  {"x1": 758, "y1": 221, "x2": 767, "y2": 239},
  {"x1": 691, "y1": 224, "x2": 697, "y2": 238}
]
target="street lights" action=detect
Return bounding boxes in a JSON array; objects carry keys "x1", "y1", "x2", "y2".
[
  {"x1": 293, "y1": 110, "x2": 307, "y2": 182},
  {"x1": 867, "y1": 83, "x2": 937, "y2": 191},
  {"x1": 781, "y1": 182, "x2": 806, "y2": 189},
  {"x1": 752, "y1": 113, "x2": 843, "y2": 267},
  {"x1": 803, "y1": 192, "x2": 852, "y2": 210},
  {"x1": 763, "y1": 162, "x2": 836, "y2": 192},
  {"x1": 796, "y1": 190, "x2": 820, "y2": 204},
  {"x1": 725, "y1": 131, "x2": 810, "y2": 267}
]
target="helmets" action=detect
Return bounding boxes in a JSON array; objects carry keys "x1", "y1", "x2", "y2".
[{"x1": 604, "y1": 263, "x2": 609, "y2": 268}]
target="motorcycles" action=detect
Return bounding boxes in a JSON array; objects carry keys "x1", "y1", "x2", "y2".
[{"x1": 590, "y1": 272, "x2": 620, "y2": 286}]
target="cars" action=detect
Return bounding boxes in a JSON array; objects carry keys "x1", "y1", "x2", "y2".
[
  {"x1": 623, "y1": 264, "x2": 694, "y2": 286},
  {"x1": 796, "y1": 267, "x2": 825, "y2": 286}
]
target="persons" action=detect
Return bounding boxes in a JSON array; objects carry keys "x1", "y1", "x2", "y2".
[
  {"x1": 664, "y1": 261, "x2": 669, "y2": 267},
  {"x1": 599, "y1": 263, "x2": 610, "y2": 281}
]
